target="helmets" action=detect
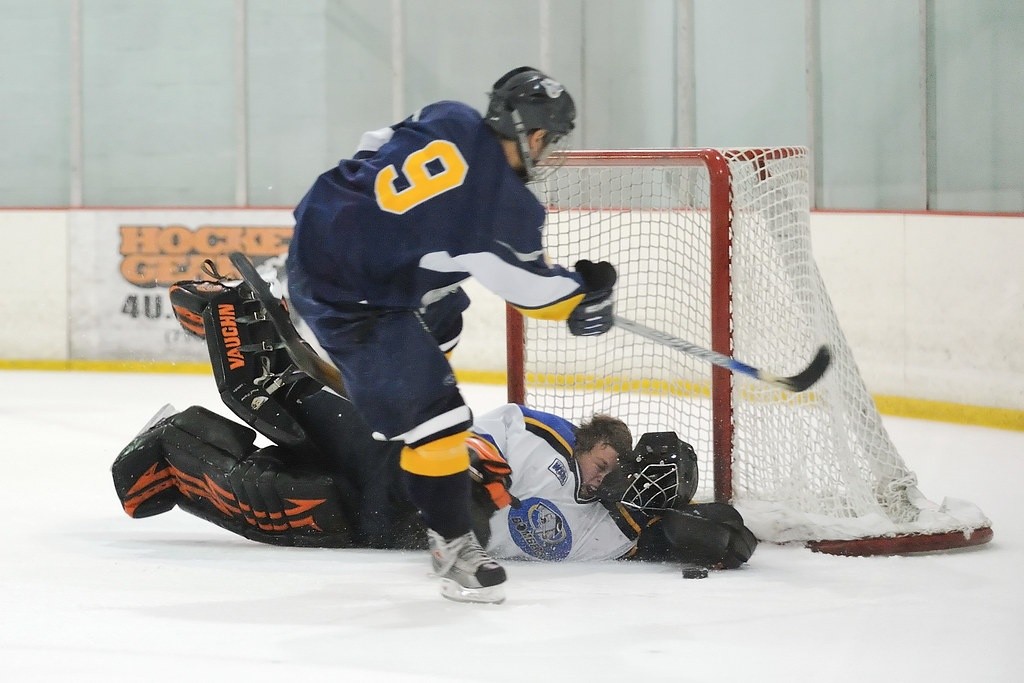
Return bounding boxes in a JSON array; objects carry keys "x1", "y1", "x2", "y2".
[
  {"x1": 483, "y1": 65, "x2": 577, "y2": 183},
  {"x1": 594, "y1": 431, "x2": 699, "y2": 514}
]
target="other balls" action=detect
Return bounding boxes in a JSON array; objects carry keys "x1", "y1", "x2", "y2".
[{"x1": 681, "y1": 565, "x2": 709, "y2": 579}]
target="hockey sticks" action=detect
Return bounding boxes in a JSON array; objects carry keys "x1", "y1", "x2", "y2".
[
  {"x1": 612, "y1": 314, "x2": 833, "y2": 394},
  {"x1": 227, "y1": 249, "x2": 348, "y2": 400}
]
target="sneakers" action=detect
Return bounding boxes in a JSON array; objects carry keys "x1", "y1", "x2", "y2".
[{"x1": 415, "y1": 508, "x2": 508, "y2": 605}]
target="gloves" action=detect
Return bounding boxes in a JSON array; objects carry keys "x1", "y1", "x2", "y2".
[{"x1": 566, "y1": 259, "x2": 617, "y2": 336}]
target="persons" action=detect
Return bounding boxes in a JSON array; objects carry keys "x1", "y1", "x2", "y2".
[
  {"x1": 288, "y1": 67, "x2": 617, "y2": 604},
  {"x1": 110, "y1": 251, "x2": 756, "y2": 566}
]
